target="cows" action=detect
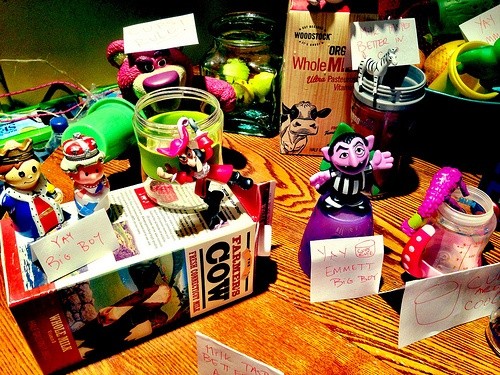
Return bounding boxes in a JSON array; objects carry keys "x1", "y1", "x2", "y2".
[{"x1": 71, "y1": 261, "x2": 185, "y2": 362}]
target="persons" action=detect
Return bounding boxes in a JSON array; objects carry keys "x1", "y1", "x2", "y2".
[
  {"x1": 156, "y1": 117, "x2": 253, "y2": 231},
  {"x1": 0, "y1": 138, "x2": 64, "y2": 239},
  {"x1": 310, "y1": 121, "x2": 394, "y2": 216},
  {"x1": 60, "y1": 133, "x2": 110, "y2": 220}
]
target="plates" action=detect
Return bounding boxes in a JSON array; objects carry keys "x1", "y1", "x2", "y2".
[{"x1": 349, "y1": 65, "x2": 426, "y2": 198}]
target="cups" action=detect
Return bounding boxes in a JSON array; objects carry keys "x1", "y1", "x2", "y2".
[
  {"x1": 60, "y1": 99, "x2": 146, "y2": 164},
  {"x1": 299, "y1": 194, "x2": 373, "y2": 278}
]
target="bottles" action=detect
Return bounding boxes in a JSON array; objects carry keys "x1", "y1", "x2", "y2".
[
  {"x1": 131, "y1": 86, "x2": 223, "y2": 209},
  {"x1": 401, "y1": 184, "x2": 500, "y2": 279},
  {"x1": 198, "y1": 12, "x2": 280, "y2": 138},
  {"x1": 44, "y1": 116, "x2": 68, "y2": 155}
]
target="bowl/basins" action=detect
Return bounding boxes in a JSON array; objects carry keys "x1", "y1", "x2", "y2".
[{"x1": 425, "y1": 86, "x2": 500, "y2": 111}]
